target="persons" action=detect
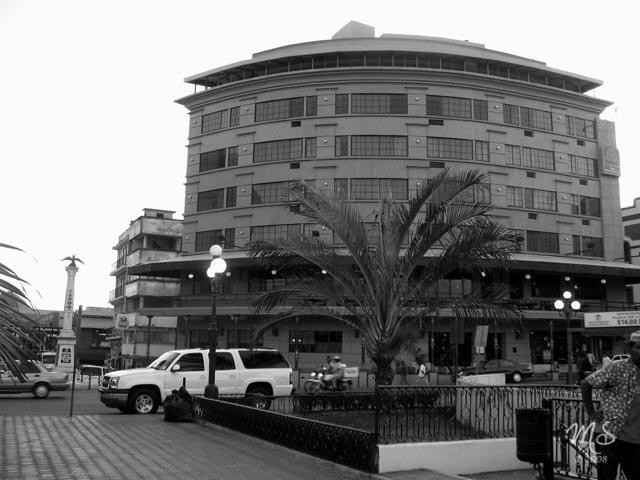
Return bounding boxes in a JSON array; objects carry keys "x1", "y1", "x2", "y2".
[{"x1": 579, "y1": 329, "x2": 639, "y2": 480}]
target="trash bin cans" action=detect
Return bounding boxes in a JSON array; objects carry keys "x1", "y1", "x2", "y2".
[{"x1": 515, "y1": 408, "x2": 550, "y2": 462}]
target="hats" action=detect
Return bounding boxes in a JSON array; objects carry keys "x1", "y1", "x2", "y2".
[
  {"x1": 333, "y1": 356, "x2": 341, "y2": 360},
  {"x1": 630, "y1": 329, "x2": 640, "y2": 343}
]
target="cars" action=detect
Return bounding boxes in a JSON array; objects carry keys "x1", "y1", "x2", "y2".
[
  {"x1": 0, "y1": 359, "x2": 73, "y2": 400},
  {"x1": 610, "y1": 354, "x2": 631, "y2": 362},
  {"x1": 451, "y1": 357, "x2": 534, "y2": 385}
]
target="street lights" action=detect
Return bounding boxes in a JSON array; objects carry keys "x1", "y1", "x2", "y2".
[
  {"x1": 553, "y1": 288, "x2": 581, "y2": 386},
  {"x1": 203, "y1": 244, "x2": 227, "y2": 400}
]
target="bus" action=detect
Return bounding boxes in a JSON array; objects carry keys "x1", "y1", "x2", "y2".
[{"x1": 36, "y1": 351, "x2": 57, "y2": 369}]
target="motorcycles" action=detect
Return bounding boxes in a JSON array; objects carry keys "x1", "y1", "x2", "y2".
[{"x1": 303, "y1": 365, "x2": 354, "y2": 394}]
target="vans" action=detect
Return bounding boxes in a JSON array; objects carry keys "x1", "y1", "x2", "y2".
[
  {"x1": 78, "y1": 364, "x2": 113, "y2": 384},
  {"x1": 96, "y1": 346, "x2": 296, "y2": 415}
]
